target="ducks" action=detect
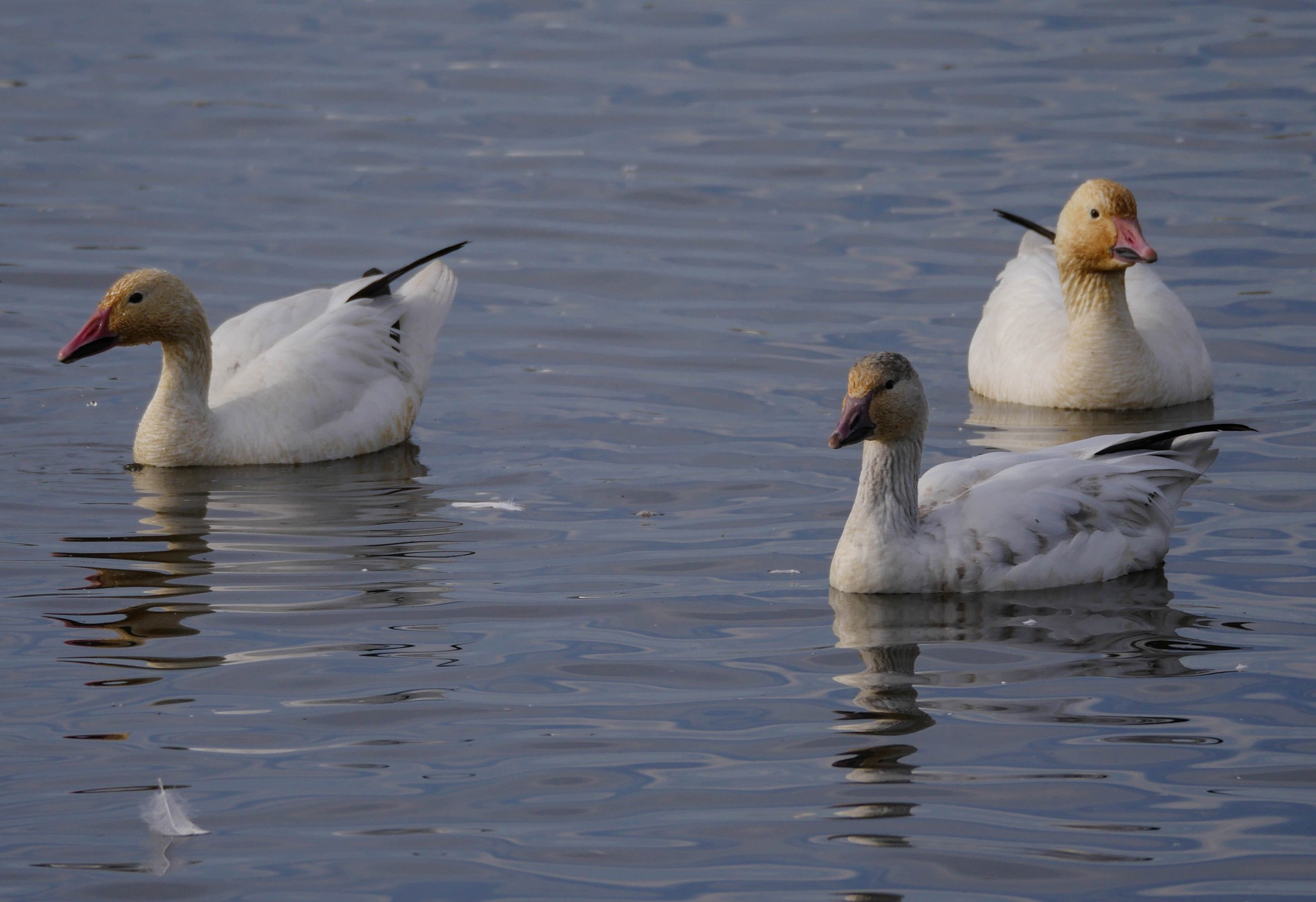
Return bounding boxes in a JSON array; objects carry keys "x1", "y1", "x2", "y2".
[
  {"x1": 828, "y1": 352, "x2": 1259, "y2": 594},
  {"x1": 57, "y1": 239, "x2": 473, "y2": 467},
  {"x1": 968, "y1": 179, "x2": 1214, "y2": 407}
]
